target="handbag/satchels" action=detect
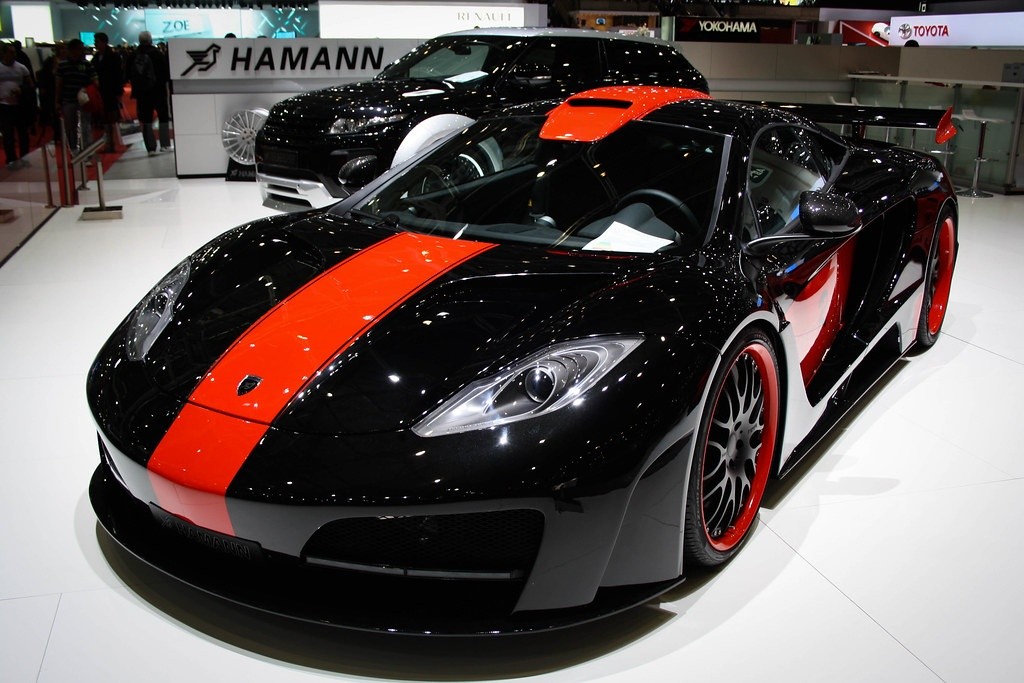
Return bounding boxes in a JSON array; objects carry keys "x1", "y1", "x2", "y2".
[{"x1": 86, "y1": 85, "x2": 104, "y2": 113}]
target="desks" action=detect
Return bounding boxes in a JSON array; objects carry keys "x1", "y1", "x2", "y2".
[{"x1": 847, "y1": 73, "x2": 1024, "y2": 196}]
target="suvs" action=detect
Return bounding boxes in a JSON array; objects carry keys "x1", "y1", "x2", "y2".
[{"x1": 254, "y1": 26, "x2": 710, "y2": 213}]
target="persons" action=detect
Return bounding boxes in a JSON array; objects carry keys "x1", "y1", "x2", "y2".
[
  {"x1": 904, "y1": 39, "x2": 919, "y2": 47},
  {"x1": 225, "y1": 33, "x2": 236, "y2": 38},
  {"x1": 0, "y1": 31, "x2": 175, "y2": 171}
]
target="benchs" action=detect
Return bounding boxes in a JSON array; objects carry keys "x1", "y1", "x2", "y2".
[{"x1": 546, "y1": 148, "x2": 717, "y2": 241}]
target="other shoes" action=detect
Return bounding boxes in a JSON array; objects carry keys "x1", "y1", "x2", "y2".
[
  {"x1": 8, "y1": 160, "x2": 15, "y2": 169},
  {"x1": 161, "y1": 147, "x2": 174, "y2": 152},
  {"x1": 21, "y1": 159, "x2": 30, "y2": 167},
  {"x1": 71, "y1": 149, "x2": 79, "y2": 157},
  {"x1": 84, "y1": 161, "x2": 92, "y2": 167},
  {"x1": 149, "y1": 151, "x2": 156, "y2": 157}
]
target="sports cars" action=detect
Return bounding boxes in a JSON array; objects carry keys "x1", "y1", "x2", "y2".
[{"x1": 85, "y1": 84, "x2": 959, "y2": 641}]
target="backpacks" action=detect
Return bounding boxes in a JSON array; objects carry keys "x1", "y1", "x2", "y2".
[{"x1": 134, "y1": 48, "x2": 156, "y2": 77}]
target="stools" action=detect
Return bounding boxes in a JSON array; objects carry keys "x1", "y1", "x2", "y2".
[
  {"x1": 830, "y1": 96, "x2": 852, "y2": 135},
  {"x1": 898, "y1": 103, "x2": 917, "y2": 149},
  {"x1": 875, "y1": 99, "x2": 891, "y2": 143},
  {"x1": 955, "y1": 109, "x2": 1010, "y2": 198},
  {"x1": 850, "y1": 96, "x2": 868, "y2": 139},
  {"x1": 925, "y1": 105, "x2": 966, "y2": 192}
]
